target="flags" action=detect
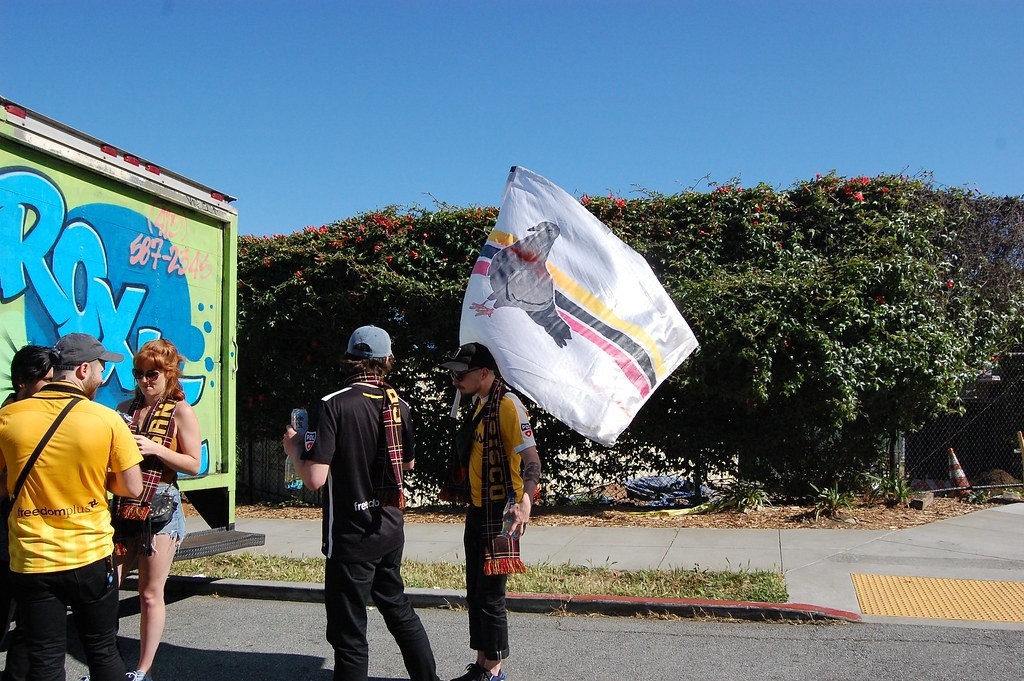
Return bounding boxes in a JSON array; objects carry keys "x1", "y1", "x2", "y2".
[{"x1": 460, "y1": 168, "x2": 700, "y2": 447}]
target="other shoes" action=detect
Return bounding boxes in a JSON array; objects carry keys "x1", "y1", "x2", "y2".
[{"x1": 124, "y1": 671, "x2": 153, "y2": 681}]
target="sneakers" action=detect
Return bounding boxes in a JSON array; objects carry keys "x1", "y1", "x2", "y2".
[
  {"x1": 471, "y1": 666, "x2": 506, "y2": 681},
  {"x1": 449, "y1": 661, "x2": 482, "y2": 681}
]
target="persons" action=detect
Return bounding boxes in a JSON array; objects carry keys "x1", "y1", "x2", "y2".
[
  {"x1": 0, "y1": 334, "x2": 144, "y2": 681},
  {"x1": 444, "y1": 343, "x2": 541, "y2": 681},
  {"x1": 80, "y1": 338, "x2": 202, "y2": 681},
  {"x1": 282, "y1": 325, "x2": 442, "y2": 681}
]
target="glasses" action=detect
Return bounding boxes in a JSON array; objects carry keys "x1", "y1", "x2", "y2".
[
  {"x1": 131, "y1": 369, "x2": 166, "y2": 381},
  {"x1": 450, "y1": 366, "x2": 483, "y2": 381}
]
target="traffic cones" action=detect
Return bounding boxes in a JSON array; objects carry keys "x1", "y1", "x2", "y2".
[{"x1": 946, "y1": 447, "x2": 972, "y2": 499}]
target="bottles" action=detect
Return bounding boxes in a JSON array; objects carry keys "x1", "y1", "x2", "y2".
[
  {"x1": 285, "y1": 455, "x2": 303, "y2": 489},
  {"x1": 502, "y1": 492, "x2": 520, "y2": 539}
]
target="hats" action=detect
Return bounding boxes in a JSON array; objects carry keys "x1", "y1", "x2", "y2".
[
  {"x1": 347, "y1": 324, "x2": 395, "y2": 359},
  {"x1": 49, "y1": 333, "x2": 125, "y2": 370},
  {"x1": 439, "y1": 342, "x2": 494, "y2": 371}
]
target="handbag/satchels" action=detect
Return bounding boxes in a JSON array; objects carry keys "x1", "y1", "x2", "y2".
[{"x1": 117, "y1": 493, "x2": 178, "y2": 534}]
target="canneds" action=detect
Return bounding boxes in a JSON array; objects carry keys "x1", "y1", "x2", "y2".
[{"x1": 290, "y1": 408, "x2": 308, "y2": 434}]
